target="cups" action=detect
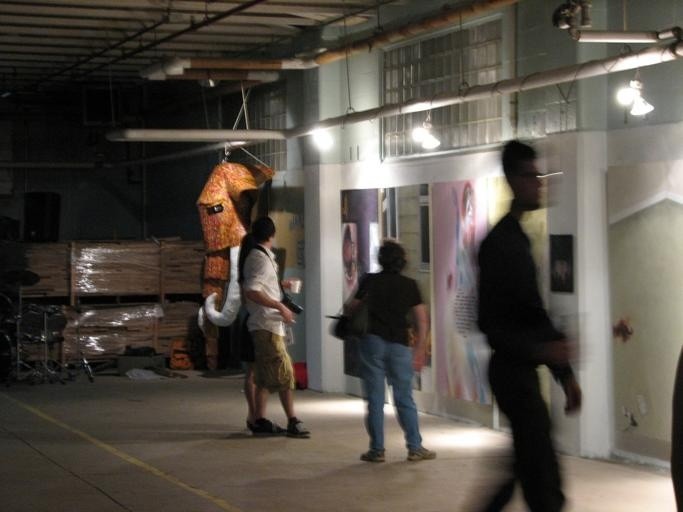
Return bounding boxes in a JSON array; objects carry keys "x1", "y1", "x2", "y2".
[{"x1": 291, "y1": 280, "x2": 302, "y2": 294}]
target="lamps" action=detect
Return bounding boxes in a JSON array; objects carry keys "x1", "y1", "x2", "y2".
[
  {"x1": 615, "y1": 69, "x2": 655, "y2": 117},
  {"x1": 412, "y1": 109, "x2": 440, "y2": 149}
]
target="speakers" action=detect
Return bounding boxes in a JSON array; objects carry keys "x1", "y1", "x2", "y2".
[{"x1": 22, "y1": 191, "x2": 62, "y2": 242}]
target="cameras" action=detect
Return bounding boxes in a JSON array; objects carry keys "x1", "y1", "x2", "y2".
[{"x1": 282, "y1": 294, "x2": 304, "y2": 314}]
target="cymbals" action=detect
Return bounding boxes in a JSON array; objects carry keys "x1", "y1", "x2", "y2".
[{"x1": 1, "y1": 269, "x2": 37, "y2": 286}]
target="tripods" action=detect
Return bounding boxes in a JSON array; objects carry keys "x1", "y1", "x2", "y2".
[{"x1": 11, "y1": 285, "x2": 95, "y2": 384}]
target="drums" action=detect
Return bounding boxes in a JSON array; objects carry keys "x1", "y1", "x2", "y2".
[
  {"x1": 0, "y1": 288, "x2": 19, "y2": 321},
  {"x1": 22, "y1": 305, "x2": 64, "y2": 346},
  {"x1": 0, "y1": 328, "x2": 22, "y2": 384}
]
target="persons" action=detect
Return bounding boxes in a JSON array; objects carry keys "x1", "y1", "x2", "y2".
[
  {"x1": 231, "y1": 224, "x2": 268, "y2": 430},
  {"x1": 340, "y1": 224, "x2": 358, "y2": 300},
  {"x1": 341, "y1": 236, "x2": 438, "y2": 462},
  {"x1": 474, "y1": 139, "x2": 584, "y2": 510},
  {"x1": 241, "y1": 214, "x2": 312, "y2": 438}
]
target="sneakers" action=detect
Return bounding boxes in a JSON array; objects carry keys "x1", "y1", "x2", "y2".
[
  {"x1": 285, "y1": 420, "x2": 310, "y2": 438},
  {"x1": 406, "y1": 448, "x2": 436, "y2": 461},
  {"x1": 245, "y1": 417, "x2": 286, "y2": 436},
  {"x1": 359, "y1": 448, "x2": 385, "y2": 462}
]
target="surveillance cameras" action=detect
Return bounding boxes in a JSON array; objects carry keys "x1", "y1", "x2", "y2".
[{"x1": 552, "y1": 0, "x2": 592, "y2": 31}]
